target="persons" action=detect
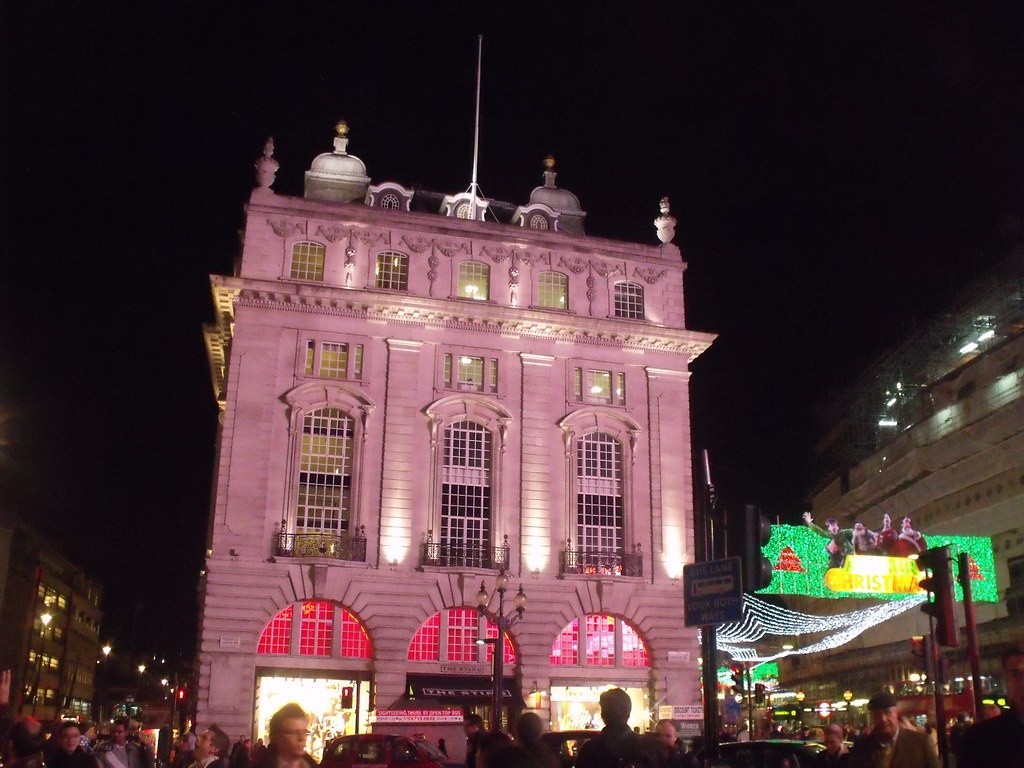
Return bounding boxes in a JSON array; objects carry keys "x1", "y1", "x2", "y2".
[
  {"x1": 953, "y1": 645, "x2": 1024, "y2": 768},
  {"x1": 464, "y1": 686, "x2": 1002, "y2": 768},
  {"x1": 0, "y1": 669, "x2": 319, "y2": 768}
]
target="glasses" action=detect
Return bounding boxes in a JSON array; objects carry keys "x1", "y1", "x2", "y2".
[{"x1": 113, "y1": 729, "x2": 126, "y2": 734}]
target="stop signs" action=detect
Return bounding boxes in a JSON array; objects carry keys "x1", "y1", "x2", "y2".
[{"x1": 817, "y1": 701, "x2": 832, "y2": 719}]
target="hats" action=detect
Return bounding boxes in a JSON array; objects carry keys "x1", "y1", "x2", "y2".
[{"x1": 867, "y1": 694, "x2": 896, "y2": 710}]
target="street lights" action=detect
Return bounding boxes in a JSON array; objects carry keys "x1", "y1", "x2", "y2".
[
  {"x1": 796, "y1": 689, "x2": 805, "y2": 742},
  {"x1": 843, "y1": 690, "x2": 852, "y2": 711},
  {"x1": 41, "y1": 594, "x2": 111, "y2": 728},
  {"x1": 476, "y1": 567, "x2": 527, "y2": 733}
]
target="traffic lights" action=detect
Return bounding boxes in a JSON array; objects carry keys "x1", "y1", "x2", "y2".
[
  {"x1": 342, "y1": 687, "x2": 353, "y2": 709},
  {"x1": 915, "y1": 546, "x2": 961, "y2": 647},
  {"x1": 177, "y1": 687, "x2": 187, "y2": 700},
  {"x1": 755, "y1": 683, "x2": 765, "y2": 704},
  {"x1": 731, "y1": 664, "x2": 744, "y2": 704}
]
